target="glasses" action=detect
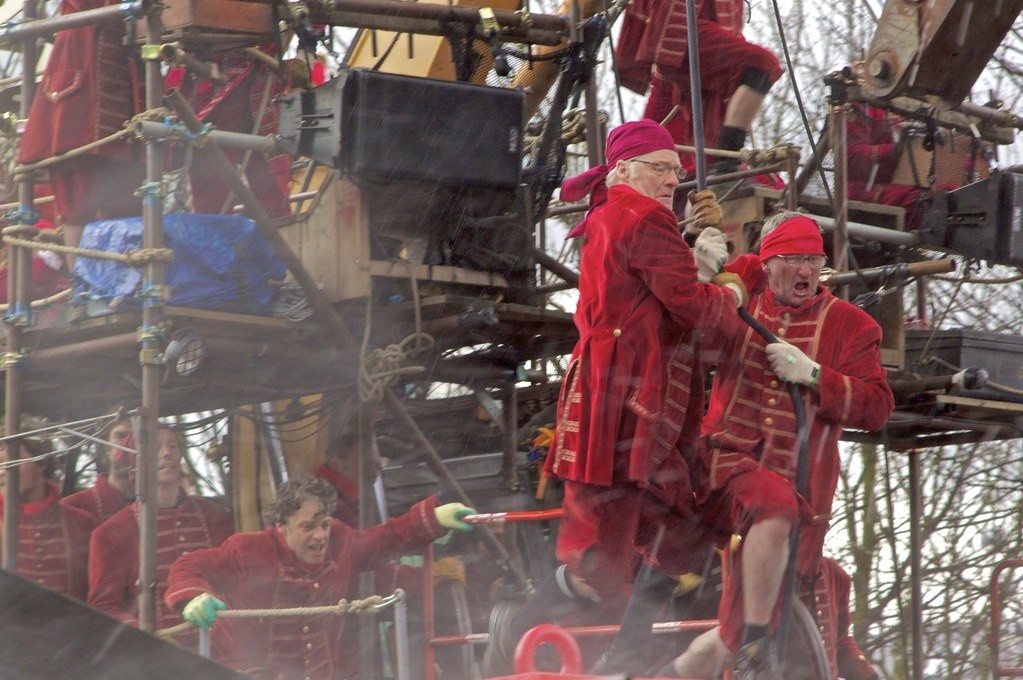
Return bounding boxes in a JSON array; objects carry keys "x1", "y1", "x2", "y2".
[
  {"x1": 777, "y1": 254, "x2": 829, "y2": 269},
  {"x1": 631, "y1": 159, "x2": 687, "y2": 179}
]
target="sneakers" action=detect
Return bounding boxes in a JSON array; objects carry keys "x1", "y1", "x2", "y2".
[{"x1": 482, "y1": 603, "x2": 522, "y2": 676}]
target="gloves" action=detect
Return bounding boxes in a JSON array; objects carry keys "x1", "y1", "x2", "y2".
[
  {"x1": 687, "y1": 189, "x2": 723, "y2": 234},
  {"x1": 693, "y1": 227, "x2": 729, "y2": 282},
  {"x1": 182, "y1": 593, "x2": 226, "y2": 633},
  {"x1": 764, "y1": 337, "x2": 821, "y2": 388},
  {"x1": 712, "y1": 272, "x2": 748, "y2": 309},
  {"x1": 281, "y1": 58, "x2": 311, "y2": 89},
  {"x1": 434, "y1": 502, "x2": 480, "y2": 533}
]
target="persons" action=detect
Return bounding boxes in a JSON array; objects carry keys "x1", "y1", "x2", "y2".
[
  {"x1": 315, "y1": 432, "x2": 468, "y2": 680},
  {"x1": 60, "y1": 405, "x2": 132, "y2": 522},
  {"x1": 0, "y1": 412, "x2": 98, "y2": 601},
  {"x1": 1, "y1": 0, "x2": 297, "y2": 330},
  {"x1": 484, "y1": 114, "x2": 747, "y2": 679},
  {"x1": 846, "y1": 58, "x2": 991, "y2": 233},
  {"x1": 652, "y1": 212, "x2": 894, "y2": 680},
  {"x1": 798, "y1": 556, "x2": 881, "y2": 680},
  {"x1": 165, "y1": 472, "x2": 478, "y2": 680},
  {"x1": 89, "y1": 422, "x2": 235, "y2": 657},
  {"x1": 610, "y1": 1, "x2": 780, "y2": 232}
]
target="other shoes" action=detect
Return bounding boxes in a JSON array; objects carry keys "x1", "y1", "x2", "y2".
[
  {"x1": 707, "y1": 159, "x2": 768, "y2": 202},
  {"x1": 66, "y1": 275, "x2": 116, "y2": 321},
  {"x1": 589, "y1": 638, "x2": 668, "y2": 677},
  {"x1": 736, "y1": 635, "x2": 785, "y2": 680}
]
